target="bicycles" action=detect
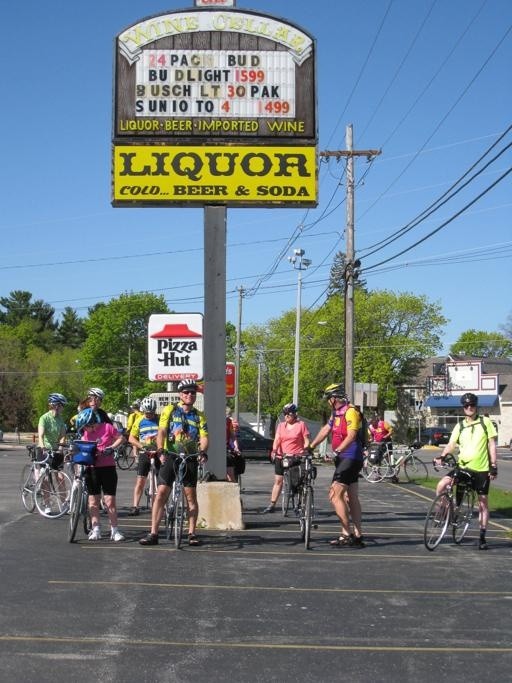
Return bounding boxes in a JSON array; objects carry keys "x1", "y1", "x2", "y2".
[
  {"x1": 362, "y1": 439, "x2": 430, "y2": 487},
  {"x1": 19, "y1": 437, "x2": 106, "y2": 543},
  {"x1": 270, "y1": 447, "x2": 324, "y2": 549},
  {"x1": 423, "y1": 453, "x2": 481, "y2": 550},
  {"x1": 113, "y1": 431, "x2": 245, "y2": 551}
]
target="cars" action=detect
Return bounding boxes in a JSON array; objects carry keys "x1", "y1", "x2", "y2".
[
  {"x1": 414, "y1": 422, "x2": 457, "y2": 448},
  {"x1": 229, "y1": 414, "x2": 285, "y2": 461}
]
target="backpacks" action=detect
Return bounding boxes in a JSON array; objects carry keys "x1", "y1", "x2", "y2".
[
  {"x1": 350, "y1": 406, "x2": 368, "y2": 448},
  {"x1": 368, "y1": 441, "x2": 386, "y2": 463}
]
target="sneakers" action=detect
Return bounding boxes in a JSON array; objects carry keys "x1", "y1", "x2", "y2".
[
  {"x1": 351, "y1": 532, "x2": 363, "y2": 545},
  {"x1": 110, "y1": 531, "x2": 125, "y2": 541},
  {"x1": 330, "y1": 534, "x2": 354, "y2": 545},
  {"x1": 139, "y1": 533, "x2": 158, "y2": 544},
  {"x1": 128, "y1": 507, "x2": 139, "y2": 515},
  {"x1": 188, "y1": 533, "x2": 199, "y2": 545},
  {"x1": 479, "y1": 539, "x2": 488, "y2": 549},
  {"x1": 264, "y1": 506, "x2": 275, "y2": 512},
  {"x1": 88, "y1": 530, "x2": 101, "y2": 541},
  {"x1": 44, "y1": 506, "x2": 52, "y2": 514}
]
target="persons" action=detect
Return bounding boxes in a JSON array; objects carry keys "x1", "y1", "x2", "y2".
[
  {"x1": 367, "y1": 412, "x2": 398, "y2": 482},
  {"x1": 262, "y1": 403, "x2": 310, "y2": 514},
  {"x1": 434, "y1": 392, "x2": 499, "y2": 551},
  {"x1": 303, "y1": 381, "x2": 365, "y2": 547},
  {"x1": 32, "y1": 379, "x2": 243, "y2": 548}
]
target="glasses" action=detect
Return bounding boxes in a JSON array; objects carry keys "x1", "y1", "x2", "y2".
[
  {"x1": 285, "y1": 412, "x2": 294, "y2": 415},
  {"x1": 181, "y1": 390, "x2": 196, "y2": 395},
  {"x1": 462, "y1": 403, "x2": 474, "y2": 408}
]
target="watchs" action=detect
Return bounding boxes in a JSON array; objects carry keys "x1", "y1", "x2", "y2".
[{"x1": 489, "y1": 460, "x2": 498, "y2": 466}]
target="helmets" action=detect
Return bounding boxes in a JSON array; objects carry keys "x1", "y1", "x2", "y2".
[
  {"x1": 322, "y1": 382, "x2": 345, "y2": 401},
  {"x1": 141, "y1": 397, "x2": 156, "y2": 413},
  {"x1": 132, "y1": 401, "x2": 140, "y2": 409},
  {"x1": 48, "y1": 392, "x2": 68, "y2": 405},
  {"x1": 283, "y1": 403, "x2": 296, "y2": 412},
  {"x1": 76, "y1": 407, "x2": 93, "y2": 431},
  {"x1": 461, "y1": 393, "x2": 477, "y2": 403},
  {"x1": 178, "y1": 378, "x2": 198, "y2": 392},
  {"x1": 88, "y1": 387, "x2": 104, "y2": 399}
]
